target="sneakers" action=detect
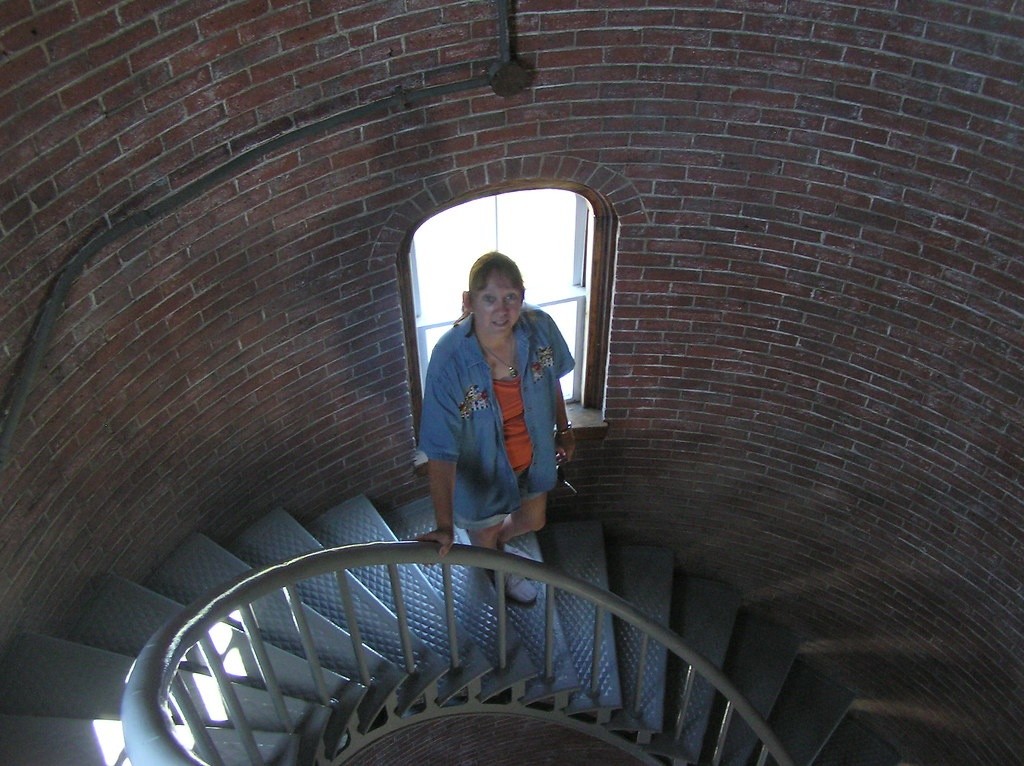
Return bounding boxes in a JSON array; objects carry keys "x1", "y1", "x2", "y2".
[
  {"x1": 500, "y1": 542, "x2": 533, "y2": 561},
  {"x1": 488, "y1": 569, "x2": 537, "y2": 603}
]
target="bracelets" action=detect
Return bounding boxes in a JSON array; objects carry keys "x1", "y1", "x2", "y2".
[{"x1": 554, "y1": 420, "x2": 572, "y2": 435}]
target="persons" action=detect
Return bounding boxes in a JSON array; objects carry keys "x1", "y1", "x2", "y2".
[{"x1": 415, "y1": 253, "x2": 576, "y2": 603}]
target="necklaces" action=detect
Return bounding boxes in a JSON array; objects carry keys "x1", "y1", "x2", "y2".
[{"x1": 477, "y1": 336, "x2": 517, "y2": 378}]
